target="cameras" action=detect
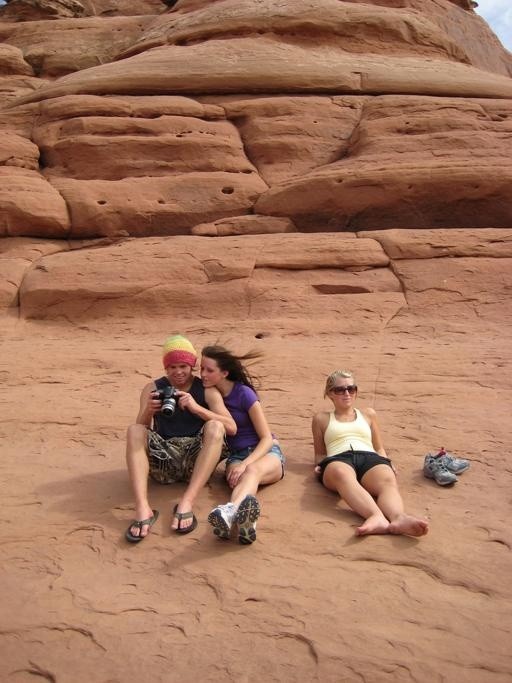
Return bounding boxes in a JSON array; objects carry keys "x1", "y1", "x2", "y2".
[{"x1": 151, "y1": 387, "x2": 181, "y2": 418}]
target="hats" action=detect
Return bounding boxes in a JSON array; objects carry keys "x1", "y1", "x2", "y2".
[{"x1": 161, "y1": 335, "x2": 198, "y2": 368}]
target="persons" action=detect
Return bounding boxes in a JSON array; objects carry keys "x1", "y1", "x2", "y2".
[
  {"x1": 125, "y1": 331, "x2": 240, "y2": 542},
  {"x1": 311, "y1": 366, "x2": 431, "y2": 538},
  {"x1": 198, "y1": 342, "x2": 285, "y2": 546}
]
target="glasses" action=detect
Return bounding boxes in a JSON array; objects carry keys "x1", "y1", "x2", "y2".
[{"x1": 332, "y1": 384, "x2": 357, "y2": 396}]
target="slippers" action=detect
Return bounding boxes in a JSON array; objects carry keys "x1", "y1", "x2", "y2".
[
  {"x1": 125, "y1": 509, "x2": 159, "y2": 543},
  {"x1": 172, "y1": 502, "x2": 198, "y2": 534}
]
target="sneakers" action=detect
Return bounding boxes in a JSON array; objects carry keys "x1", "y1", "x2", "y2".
[
  {"x1": 209, "y1": 504, "x2": 235, "y2": 539},
  {"x1": 234, "y1": 494, "x2": 259, "y2": 544},
  {"x1": 435, "y1": 450, "x2": 470, "y2": 475},
  {"x1": 423, "y1": 455, "x2": 457, "y2": 486}
]
